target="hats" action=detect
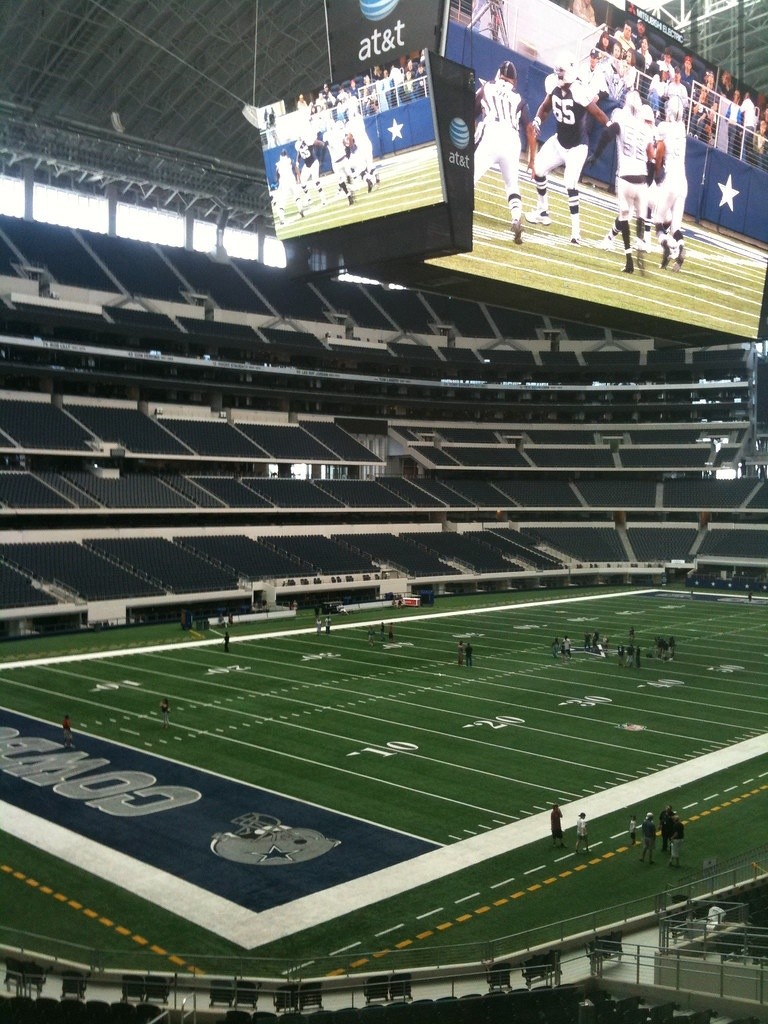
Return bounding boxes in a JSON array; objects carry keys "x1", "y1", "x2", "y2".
[
  {"x1": 645, "y1": 813, "x2": 653, "y2": 820},
  {"x1": 590, "y1": 49, "x2": 599, "y2": 58},
  {"x1": 638, "y1": 19, "x2": 646, "y2": 28},
  {"x1": 499, "y1": 62, "x2": 518, "y2": 80},
  {"x1": 659, "y1": 63, "x2": 670, "y2": 72}
]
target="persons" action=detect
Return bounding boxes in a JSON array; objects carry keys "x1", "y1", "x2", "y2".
[
  {"x1": 458, "y1": 641, "x2": 473, "y2": 669},
  {"x1": 551, "y1": 803, "x2": 566, "y2": 848},
  {"x1": 392, "y1": 595, "x2": 404, "y2": 609},
  {"x1": 63, "y1": 713, "x2": 76, "y2": 749},
  {"x1": 265, "y1": 50, "x2": 433, "y2": 224},
  {"x1": 575, "y1": 812, "x2": 591, "y2": 852},
  {"x1": 218, "y1": 613, "x2": 233, "y2": 628},
  {"x1": 367, "y1": 622, "x2": 394, "y2": 647},
  {"x1": 628, "y1": 805, "x2": 684, "y2": 867},
  {"x1": 314, "y1": 605, "x2": 320, "y2": 624},
  {"x1": 551, "y1": 626, "x2": 676, "y2": 670},
  {"x1": 159, "y1": 698, "x2": 171, "y2": 729},
  {"x1": 316, "y1": 616, "x2": 331, "y2": 637},
  {"x1": 475, "y1": 0, "x2": 768, "y2": 274},
  {"x1": 224, "y1": 632, "x2": 230, "y2": 653}
]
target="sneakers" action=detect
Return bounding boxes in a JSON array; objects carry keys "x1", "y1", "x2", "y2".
[
  {"x1": 571, "y1": 233, "x2": 580, "y2": 244},
  {"x1": 525, "y1": 211, "x2": 551, "y2": 226}
]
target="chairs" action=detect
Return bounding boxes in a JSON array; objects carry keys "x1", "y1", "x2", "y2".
[
  {"x1": 0, "y1": 876, "x2": 768, "y2": 1024},
  {"x1": 0, "y1": 398, "x2": 768, "y2": 617}
]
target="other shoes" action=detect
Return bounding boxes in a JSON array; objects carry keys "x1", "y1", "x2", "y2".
[
  {"x1": 673, "y1": 245, "x2": 686, "y2": 270},
  {"x1": 650, "y1": 860, "x2": 655, "y2": 865},
  {"x1": 602, "y1": 237, "x2": 613, "y2": 252},
  {"x1": 513, "y1": 221, "x2": 523, "y2": 244},
  {"x1": 659, "y1": 252, "x2": 672, "y2": 269},
  {"x1": 586, "y1": 849, "x2": 592, "y2": 853},
  {"x1": 621, "y1": 265, "x2": 635, "y2": 274},
  {"x1": 656, "y1": 112, "x2": 661, "y2": 120}
]
